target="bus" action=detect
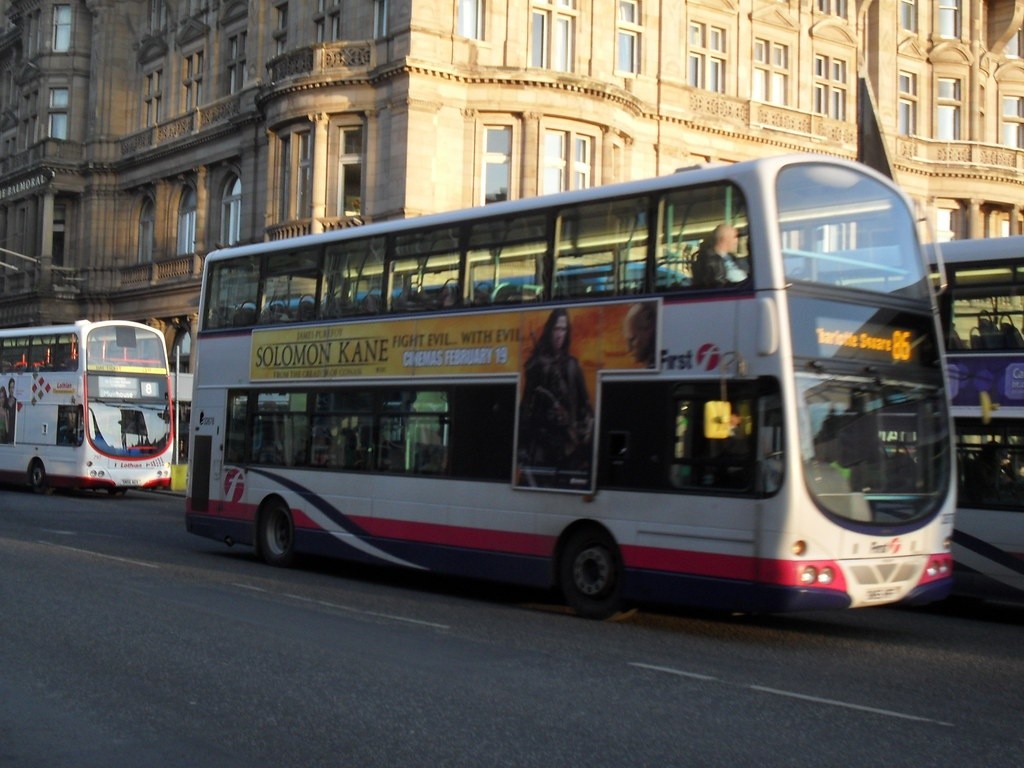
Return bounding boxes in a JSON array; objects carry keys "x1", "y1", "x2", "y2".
[
  {"x1": 0, "y1": 319, "x2": 174, "y2": 496},
  {"x1": 238, "y1": 236, "x2": 1024, "y2": 606},
  {"x1": 187, "y1": 155, "x2": 957, "y2": 621}
]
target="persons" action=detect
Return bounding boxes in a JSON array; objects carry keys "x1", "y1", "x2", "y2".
[
  {"x1": 621, "y1": 301, "x2": 657, "y2": 368},
  {"x1": 693, "y1": 223, "x2": 752, "y2": 292},
  {"x1": 0, "y1": 378, "x2": 17, "y2": 444},
  {"x1": 516, "y1": 307, "x2": 595, "y2": 491}
]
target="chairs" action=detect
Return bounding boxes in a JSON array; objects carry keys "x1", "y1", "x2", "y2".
[
  {"x1": 210, "y1": 273, "x2": 647, "y2": 332},
  {"x1": 957, "y1": 450, "x2": 1015, "y2": 500},
  {"x1": 948, "y1": 308, "x2": 1024, "y2": 353},
  {"x1": 237, "y1": 433, "x2": 752, "y2": 496}
]
